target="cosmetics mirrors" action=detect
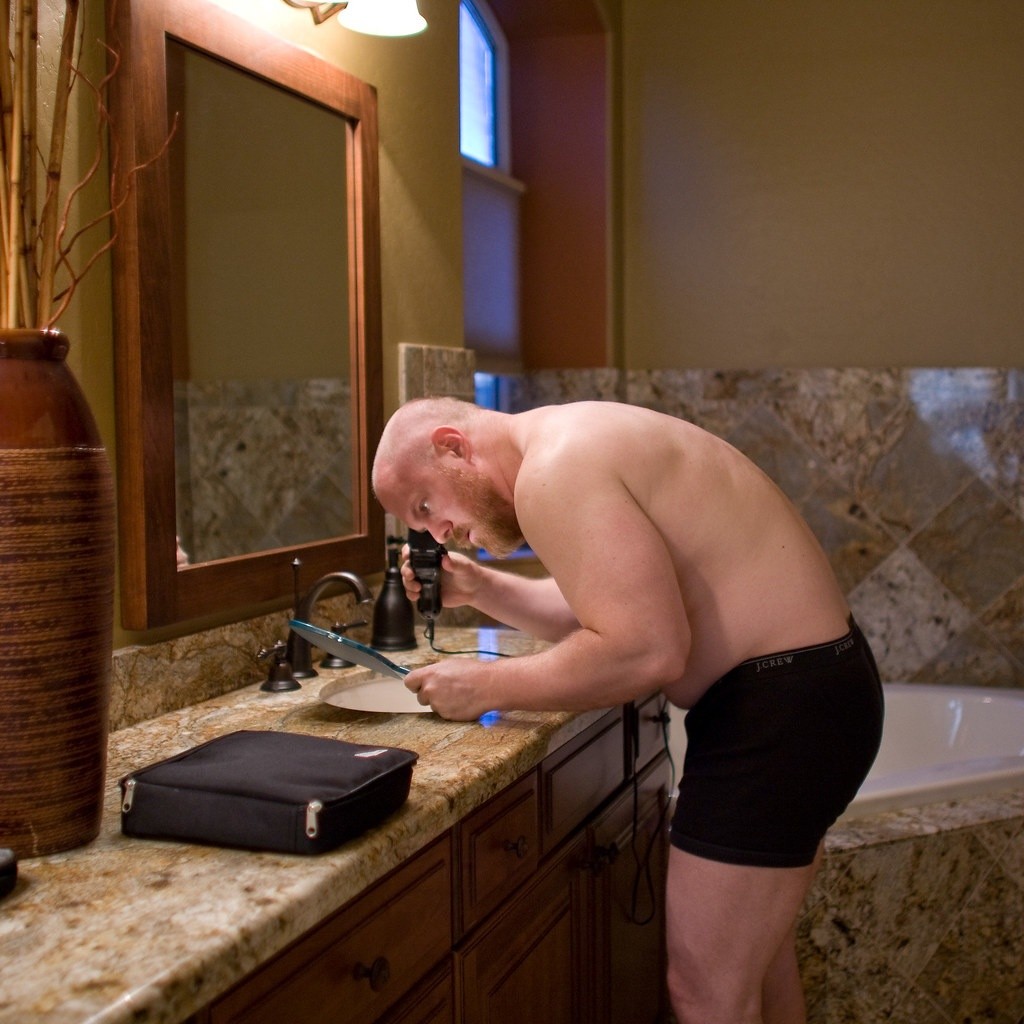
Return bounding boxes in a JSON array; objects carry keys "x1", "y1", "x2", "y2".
[{"x1": 287, "y1": 619, "x2": 412, "y2": 684}]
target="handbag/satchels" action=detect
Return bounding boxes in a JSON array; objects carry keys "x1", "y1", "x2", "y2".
[{"x1": 117, "y1": 730, "x2": 420, "y2": 858}]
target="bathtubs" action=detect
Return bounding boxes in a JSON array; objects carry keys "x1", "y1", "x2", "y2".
[{"x1": 668, "y1": 676, "x2": 1024, "y2": 856}]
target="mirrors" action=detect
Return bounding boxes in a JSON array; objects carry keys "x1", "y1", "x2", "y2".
[{"x1": 102, "y1": 0, "x2": 389, "y2": 630}]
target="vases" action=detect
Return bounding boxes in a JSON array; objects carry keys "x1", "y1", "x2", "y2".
[{"x1": 1, "y1": 324, "x2": 110, "y2": 857}]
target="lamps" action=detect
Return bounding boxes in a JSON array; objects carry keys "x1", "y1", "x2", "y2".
[{"x1": 285, "y1": 0, "x2": 427, "y2": 37}]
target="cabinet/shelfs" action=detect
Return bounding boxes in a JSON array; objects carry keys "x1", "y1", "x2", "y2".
[{"x1": 193, "y1": 678, "x2": 677, "y2": 1024}]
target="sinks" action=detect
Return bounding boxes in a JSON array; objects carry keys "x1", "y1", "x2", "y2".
[{"x1": 318, "y1": 659, "x2": 436, "y2": 716}]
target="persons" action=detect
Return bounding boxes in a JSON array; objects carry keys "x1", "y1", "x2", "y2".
[
  {"x1": 176, "y1": 536, "x2": 189, "y2": 569},
  {"x1": 371, "y1": 395, "x2": 884, "y2": 1024}
]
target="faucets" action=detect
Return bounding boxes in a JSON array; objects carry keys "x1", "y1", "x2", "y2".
[{"x1": 289, "y1": 570, "x2": 374, "y2": 681}]
